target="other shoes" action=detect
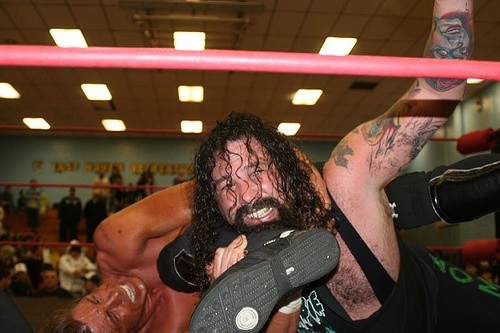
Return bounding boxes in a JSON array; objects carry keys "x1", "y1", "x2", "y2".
[{"x1": 189, "y1": 229, "x2": 341, "y2": 332}]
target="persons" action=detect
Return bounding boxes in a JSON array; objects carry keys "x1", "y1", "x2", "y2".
[
  {"x1": 191, "y1": 0, "x2": 500, "y2": 333},
  {"x1": 172, "y1": 164, "x2": 188, "y2": 185},
  {"x1": 36, "y1": 151, "x2": 499, "y2": 333},
  {"x1": 0, "y1": 159, "x2": 154, "y2": 301}
]
